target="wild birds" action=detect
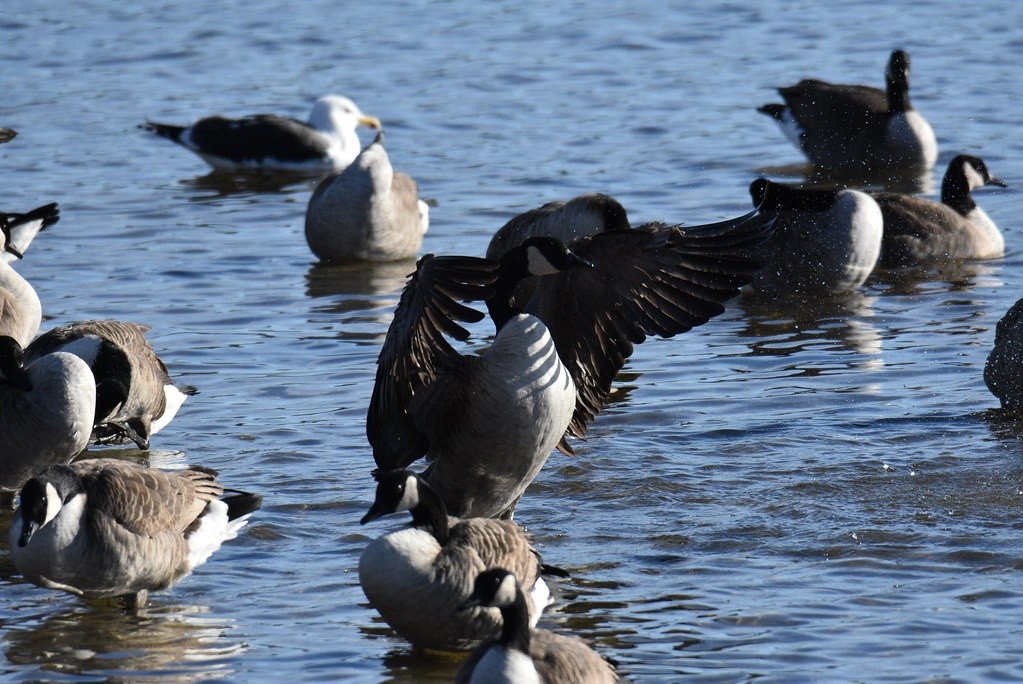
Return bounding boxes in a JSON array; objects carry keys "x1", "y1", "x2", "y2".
[
  {"x1": 414, "y1": 310, "x2": 577, "y2": 522},
  {"x1": 743, "y1": 153, "x2": 1008, "y2": 306},
  {"x1": 451, "y1": 566, "x2": 623, "y2": 684},
  {"x1": 304, "y1": 131, "x2": 431, "y2": 264},
  {"x1": 133, "y1": 94, "x2": 383, "y2": 174},
  {"x1": 364, "y1": 178, "x2": 799, "y2": 469},
  {"x1": 6, "y1": 456, "x2": 263, "y2": 613},
  {"x1": 983, "y1": 296, "x2": 1023, "y2": 414},
  {"x1": 358, "y1": 468, "x2": 572, "y2": 656},
  {"x1": 0, "y1": 128, "x2": 200, "y2": 513},
  {"x1": 755, "y1": 47, "x2": 939, "y2": 177}
]
